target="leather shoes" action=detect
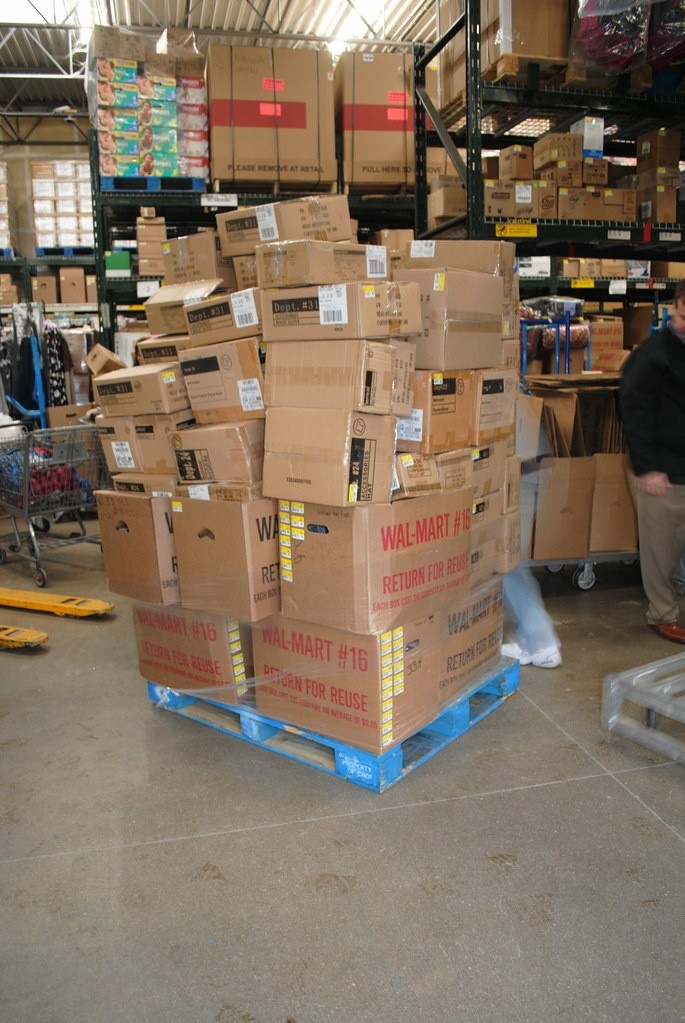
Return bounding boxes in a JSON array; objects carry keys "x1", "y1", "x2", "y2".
[{"x1": 649, "y1": 620, "x2": 685, "y2": 643}]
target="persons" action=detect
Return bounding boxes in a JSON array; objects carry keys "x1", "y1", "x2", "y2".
[
  {"x1": 96, "y1": 57, "x2": 118, "y2": 176},
  {"x1": 138, "y1": 76, "x2": 155, "y2": 176},
  {"x1": 502, "y1": 400, "x2": 562, "y2": 669},
  {"x1": 180, "y1": 84, "x2": 189, "y2": 101},
  {"x1": 620, "y1": 279, "x2": 685, "y2": 644}
]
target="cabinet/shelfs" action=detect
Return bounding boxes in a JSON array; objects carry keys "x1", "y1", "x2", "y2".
[
  {"x1": 0, "y1": 256, "x2": 99, "y2": 325},
  {"x1": 88, "y1": 129, "x2": 414, "y2": 362},
  {"x1": 412, "y1": 0, "x2": 685, "y2": 590}
]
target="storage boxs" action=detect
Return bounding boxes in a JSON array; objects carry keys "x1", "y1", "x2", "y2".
[{"x1": 0, "y1": 0, "x2": 685, "y2": 757}]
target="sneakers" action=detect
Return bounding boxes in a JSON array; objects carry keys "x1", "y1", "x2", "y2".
[{"x1": 502, "y1": 641, "x2": 562, "y2": 668}]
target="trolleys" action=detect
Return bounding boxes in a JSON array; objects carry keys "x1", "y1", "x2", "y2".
[{"x1": 0, "y1": 422, "x2": 114, "y2": 590}]
